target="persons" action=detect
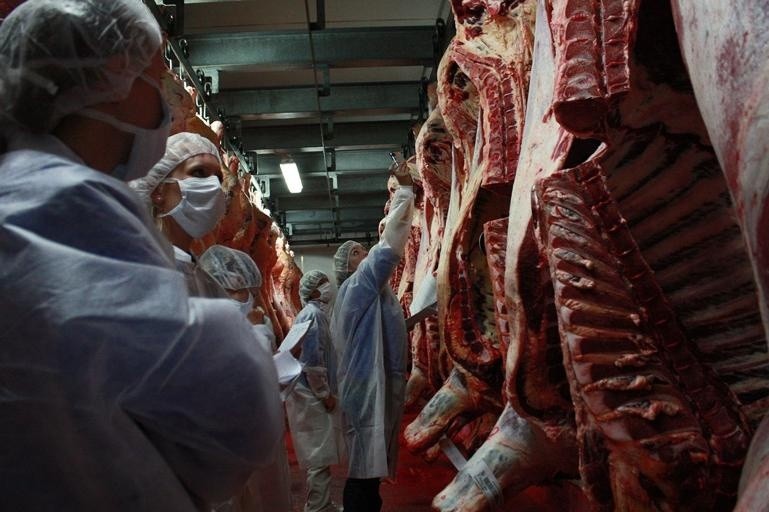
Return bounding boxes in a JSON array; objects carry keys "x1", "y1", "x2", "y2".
[
  {"x1": 197, "y1": 241, "x2": 283, "y2": 360},
  {"x1": 0, "y1": 0, "x2": 297, "y2": 512},
  {"x1": 135, "y1": 122, "x2": 235, "y2": 298},
  {"x1": 329, "y1": 159, "x2": 418, "y2": 512},
  {"x1": 281, "y1": 269, "x2": 343, "y2": 512}
]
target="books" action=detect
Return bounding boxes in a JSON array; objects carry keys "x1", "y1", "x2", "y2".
[{"x1": 271, "y1": 319, "x2": 313, "y2": 385}]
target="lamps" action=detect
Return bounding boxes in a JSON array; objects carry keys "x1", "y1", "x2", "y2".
[{"x1": 279, "y1": 156, "x2": 304, "y2": 195}]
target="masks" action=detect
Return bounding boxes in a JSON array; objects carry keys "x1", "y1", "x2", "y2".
[
  {"x1": 123, "y1": 90, "x2": 174, "y2": 185},
  {"x1": 167, "y1": 174, "x2": 228, "y2": 241},
  {"x1": 233, "y1": 291, "x2": 255, "y2": 318},
  {"x1": 317, "y1": 281, "x2": 333, "y2": 303}
]
